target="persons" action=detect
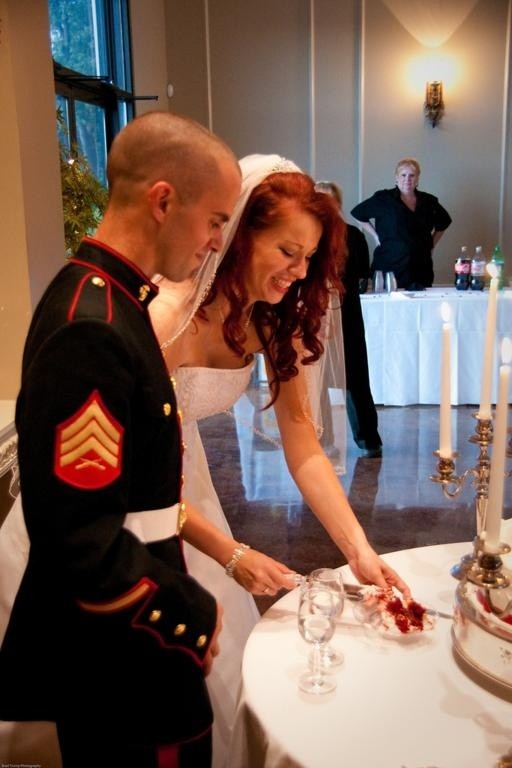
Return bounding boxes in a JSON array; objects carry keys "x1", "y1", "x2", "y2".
[
  {"x1": 349, "y1": 160, "x2": 452, "y2": 291},
  {"x1": 0, "y1": 155, "x2": 410, "y2": 767},
  {"x1": 313, "y1": 179, "x2": 383, "y2": 458},
  {"x1": 0, "y1": 113, "x2": 243, "y2": 767}
]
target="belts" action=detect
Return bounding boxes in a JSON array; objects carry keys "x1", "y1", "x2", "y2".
[{"x1": 123, "y1": 501, "x2": 190, "y2": 548}]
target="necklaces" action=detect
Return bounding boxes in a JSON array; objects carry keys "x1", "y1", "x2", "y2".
[{"x1": 214, "y1": 288, "x2": 256, "y2": 344}]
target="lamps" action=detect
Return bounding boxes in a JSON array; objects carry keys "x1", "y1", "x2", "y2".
[{"x1": 408, "y1": 46, "x2": 461, "y2": 129}]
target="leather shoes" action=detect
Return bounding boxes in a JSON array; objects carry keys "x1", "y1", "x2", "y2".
[
  {"x1": 323, "y1": 445, "x2": 341, "y2": 458},
  {"x1": 362, "y1": 447, "x2": 384, "y2": 458}
]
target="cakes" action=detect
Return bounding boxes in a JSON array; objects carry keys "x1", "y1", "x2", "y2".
[{"x1": 360, "y1": 583, "x2": 428, "y2": 634}]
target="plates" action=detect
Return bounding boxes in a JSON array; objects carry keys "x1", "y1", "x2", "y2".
[{"x1": 351, "y1": 595, "x2": 439, "y2": 636}]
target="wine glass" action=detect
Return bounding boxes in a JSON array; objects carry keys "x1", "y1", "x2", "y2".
[{"x1": 296, "y1": 568, "x2": 348, "y2": 695}]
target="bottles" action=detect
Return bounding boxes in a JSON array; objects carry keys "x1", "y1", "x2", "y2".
[
  {"x1": 454, "y1": 245, "x2": 504, "y2": 291},
  {"x1": 373, "y1": 269, "x2": 398, "y2": 293}
]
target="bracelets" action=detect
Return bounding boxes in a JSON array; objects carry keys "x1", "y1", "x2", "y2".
[{"x1": 225, "y1": 542, "x2": 249, "y2": 578}]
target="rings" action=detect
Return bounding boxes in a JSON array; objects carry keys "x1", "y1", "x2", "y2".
[{"x1": 263, "y1": 587, "x2": 270, "y2": 593}]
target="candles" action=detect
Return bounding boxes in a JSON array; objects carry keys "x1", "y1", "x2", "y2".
[
  {"x1": 480, "y1": 262, "x2": 498, "y2": 420},
  {"x1": 438, "y1": 301, "x2": 453, "y2": 458},
  {"x1": 486, "y1": 338, "x2": 512, "y2": 552}
]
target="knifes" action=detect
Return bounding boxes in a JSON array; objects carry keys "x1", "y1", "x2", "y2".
[{"x1": 285, "y1": 572, "x2": 453, "y2": 620}]
